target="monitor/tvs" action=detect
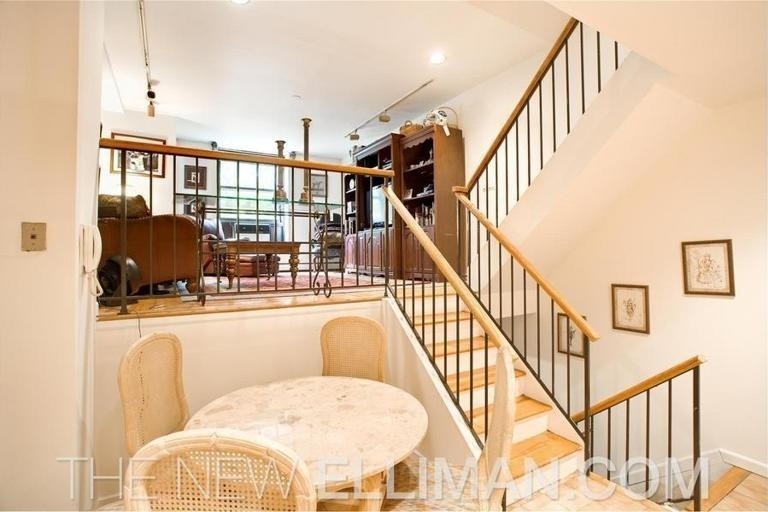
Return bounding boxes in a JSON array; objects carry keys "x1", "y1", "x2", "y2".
[{"x1": 363, "y1": 182, "x2": 392, "y2": 227}]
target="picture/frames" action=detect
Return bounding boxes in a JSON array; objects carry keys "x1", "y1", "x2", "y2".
[
  {"x1": 681, "y1": 238, "x2": 735, "y2": 296},
  {"x1": 611, "y1": 283, "x2": 650, "y2": 334},
  {"x1": 109, "y1": 132, "x2": 166, "y2": 178},
  {"x1": 184, "y1": 164, "x2": 207, "y2": 190},
  {"x1": 557, "y1": 312, "x2": 586, "y2": 358}
]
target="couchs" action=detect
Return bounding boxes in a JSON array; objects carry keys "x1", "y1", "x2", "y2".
[
  {"x1": 193, "y1": 214, "x2": 280, "y2": 277},
  {"x1": 97, "y1": 193, "x2": 199, "y2": 306}
]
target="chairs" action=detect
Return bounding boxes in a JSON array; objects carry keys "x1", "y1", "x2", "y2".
[
  {"x1": 116, "y1": 331, "x2": 190, "y2": 452},
  {"x1": 320, "y1": 315, "x2": 386, "y2": 386},
  {"x1": 124, "y1": 429, "x2": 318, "y2": 512}
]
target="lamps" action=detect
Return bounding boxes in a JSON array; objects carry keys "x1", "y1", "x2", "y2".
[
  {"x1": 350, "y1": 135, "x2": 360, "y2": 141},
  {"x1": 379, "y1": 115, "x2": 391, "y2": 122}
]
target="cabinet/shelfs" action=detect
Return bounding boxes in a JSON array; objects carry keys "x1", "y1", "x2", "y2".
[
  {"x1": 343, "y1": 174, "x2": 357, "y2": 272},
  {"x1": 400, "y1": 130, "x2": 437, "y2": 280},
  {"x1": 356, "y1": 140, "x2": 392, "y2": 276}
]
